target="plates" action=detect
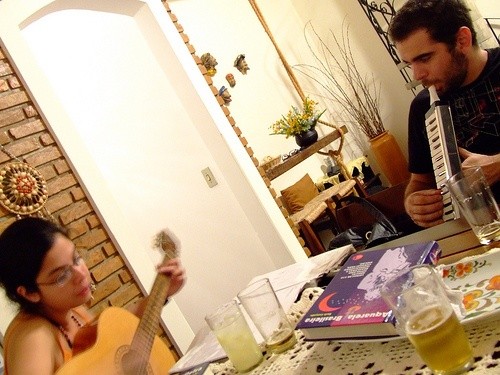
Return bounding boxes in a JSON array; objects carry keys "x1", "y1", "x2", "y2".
[{"x1": 423, "y1": 248, "x2": 500, "y2": 329}]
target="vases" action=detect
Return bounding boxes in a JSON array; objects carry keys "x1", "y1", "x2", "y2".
[
  {"x1": 294, "y1": 130, "x2": 318, "y2": 147},
  {"x1": 369, "y1": 131, "x2": 410, "y2": 186}
]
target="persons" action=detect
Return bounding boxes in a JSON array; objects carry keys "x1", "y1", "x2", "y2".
[
  {"x1": 0, "y1": 215, "x2": 186, "y2": 375},
  {"x1": 387, "y1": 0, "x2": 500, "y2": 228}
]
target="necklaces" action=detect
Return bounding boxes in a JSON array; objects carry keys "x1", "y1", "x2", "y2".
[{"x1": 57, "y1": 315, "x2": 83, "y2": 349}]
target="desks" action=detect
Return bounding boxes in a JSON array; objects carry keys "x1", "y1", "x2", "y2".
[
  {"x1": 291, "y1": 177, "x2": 368, "y2": 253},
  {"x1": 174, "y1": 205, "x2": 500, "y2": 375}
]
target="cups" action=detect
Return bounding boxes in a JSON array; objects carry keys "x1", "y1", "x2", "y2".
[
  {"x1": 380, "y1": 265, "x2": 476, "y2": 374},
  {"x1": 446, "y1": 166, "x2": 500, "y2": 244},
  {"x1": 237, "y1": 279, "x2": 298, "y2": 354},
  {"x1": 204, "y1": 299, "x2": 264, "y2": 371}
]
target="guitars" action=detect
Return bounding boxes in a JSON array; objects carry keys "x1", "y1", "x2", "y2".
[{"x1": 53, "y1": 232, "x2": 177, "y2": 375}]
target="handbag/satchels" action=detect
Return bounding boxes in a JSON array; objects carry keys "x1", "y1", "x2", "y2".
[{"x1": 325, "y1": 196, "x2": 420, "y2": 250}]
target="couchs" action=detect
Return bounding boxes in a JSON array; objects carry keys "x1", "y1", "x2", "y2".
[{"x1": 280, "y1": 156, "x2": 367, "y2": 214}]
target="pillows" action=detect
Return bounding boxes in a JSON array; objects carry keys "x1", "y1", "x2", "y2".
[{"x1": 281, "y1": 173, "x2": 319, "y2": 212}]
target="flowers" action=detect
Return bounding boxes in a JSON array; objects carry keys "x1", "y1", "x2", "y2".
[{"x1": 269, "y1": 97, "x2": 326, "y2": 136}]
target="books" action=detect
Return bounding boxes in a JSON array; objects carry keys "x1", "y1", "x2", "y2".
[
  {"x1": 167, "y1": 244, "x2": 357, "y2": 375},
  {"x1": 294, "y1": 240, "x2": 442, "y2": 341}
]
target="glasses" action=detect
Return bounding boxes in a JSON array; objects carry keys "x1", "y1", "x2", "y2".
[{"x1": 37, "y1": 255, "x2": 84, "y2": 288}]
